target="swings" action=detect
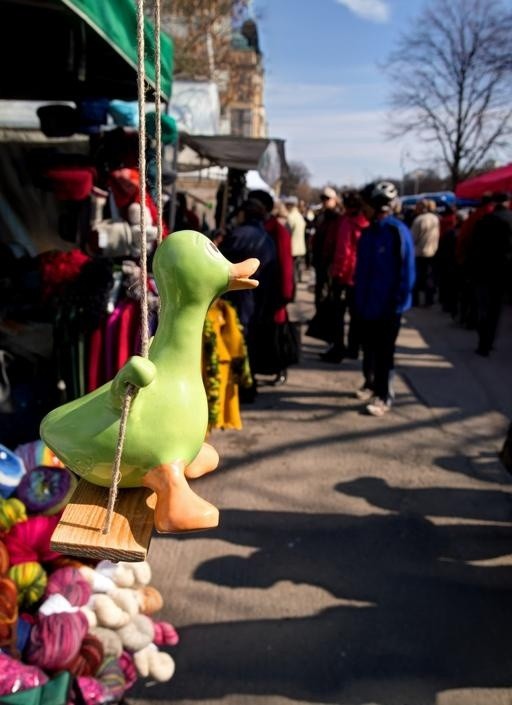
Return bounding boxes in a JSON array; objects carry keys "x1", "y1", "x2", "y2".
[{"x1": 51, "y1": 0, "x2": 162, "y2": 562}]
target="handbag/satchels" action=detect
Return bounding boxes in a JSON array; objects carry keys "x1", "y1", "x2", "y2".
[
  {"x1": 257, "y1": 321, "x2": 300, "y2": 375},
  {"x1": 305, "y1": 298, "x2": 346, "y2": 343}
]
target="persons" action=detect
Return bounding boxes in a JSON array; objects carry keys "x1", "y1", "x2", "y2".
[{"x1": 87, "y1": 129, "x2": 512, "y2": 481}]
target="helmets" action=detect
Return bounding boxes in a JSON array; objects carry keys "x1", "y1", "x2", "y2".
[{"x1": 360, "y1": 182, "x2": 398, "y2": 202}]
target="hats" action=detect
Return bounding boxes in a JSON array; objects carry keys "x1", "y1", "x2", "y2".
[{"x1": 320, "y1": 187, "x2": 336, "y2": 198}]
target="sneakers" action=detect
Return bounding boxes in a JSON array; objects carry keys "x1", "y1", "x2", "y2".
[{"x1": 356, "y1": 386, "x2": 392, "y2": 416}]
